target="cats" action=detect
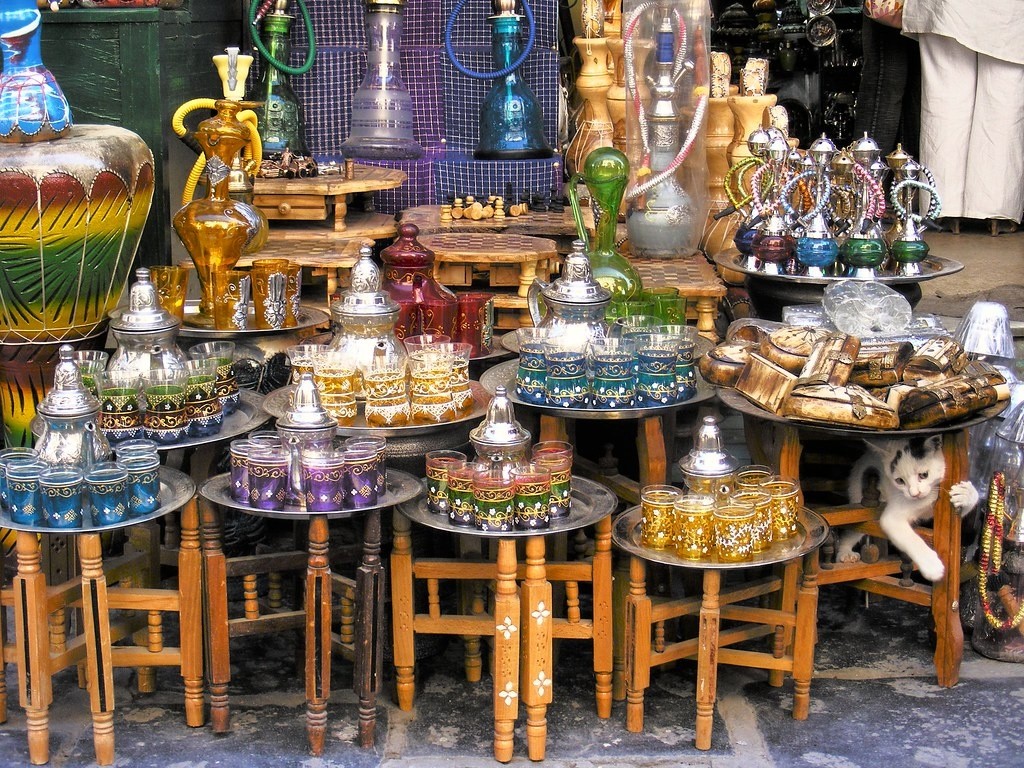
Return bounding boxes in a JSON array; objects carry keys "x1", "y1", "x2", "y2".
[{"x1": 835, "y1": 433, "x2": 978, "y2": 582}]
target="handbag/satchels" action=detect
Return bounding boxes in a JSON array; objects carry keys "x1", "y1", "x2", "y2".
[{"x1": 862, "y1": 0, "x2": 903, "y2": 28}]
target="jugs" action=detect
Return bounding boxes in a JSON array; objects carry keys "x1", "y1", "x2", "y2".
[
  {"x1": 379, "y1": 223, "x2": 459, "y2": 356},
  {"x1": 330, "y1": 244, "x2": 409, "y2": 371},
  {"x1": 678, "y1": 416, "x2": 743, "y2": 504},
  {"x1": 29, "y1": 345, "x2": 111, "y2": 470},
  {"x1": 106, "y1": 266, "x2": 187, "y2": 400},
  {"x1": 467, "y1": 384, "x2": 532, "y2": 478},
  {"x1": 527, "y1": 239, "x2": 614, "y2": 352}
]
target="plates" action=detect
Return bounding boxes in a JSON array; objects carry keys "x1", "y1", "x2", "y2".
[{"x1": 275, "y1": 373, "x2": 338, "y2": 505}]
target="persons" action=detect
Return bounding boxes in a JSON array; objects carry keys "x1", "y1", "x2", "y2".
[{"x1": 899, "y1": 0, "x2": 1024, "y2": 236}]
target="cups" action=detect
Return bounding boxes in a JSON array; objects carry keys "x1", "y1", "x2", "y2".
[
  {"x1": 288, "y1": 334, "x2": 472, "y2": 428},
  {"x1": 640, "y1": 463, "x2": 802, "y2": 562},
  {"x1": 514, "y1": 286, "x2": 700, "y2": 407},
  {"x1": 68, "y1": 341, "x2": 236, "y2": 447},
  {"x1": 149, "y1": 257, "x2": 303, "y2": 332},
  {"x1": 424, "y1": 438, "x2": 575, "y2": 533},
  {"x1": 228, "y1": 430, "x2": 386, "y2": 512},
  {"x1": 395, "y1": 292, "x2": 495, "y2": 359},
  {"x1": 0, "y1": 439, "x2": 159, "y2": 526}
]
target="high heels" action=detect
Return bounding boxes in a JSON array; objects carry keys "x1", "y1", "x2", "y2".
[
  {"x1": 991, "y1": 218, "x2": 1018, "y2": 236},
  {"x1": 946, "y1": 216, "x2": 960, "y2": 234}
]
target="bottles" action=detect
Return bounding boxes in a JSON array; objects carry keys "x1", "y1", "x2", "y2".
[
  {"x1": 250, "y1": 17, "x2": 310, "y2": 159},
  {"x1": 625, "y1": 62, "x2": 699, "y2": 258},
  {"x1": 568, "y1": 147, "x2": 644, "y2": 320},
  {"x1": 471, "y1": 19, "x2": 555, "y2": 158},
  {"x1": 173, "y1": 101, "x2": 269, "y2": 329},
  {"x1": 340, "y1": 6, "x2": 420, "y2": 158}
]
